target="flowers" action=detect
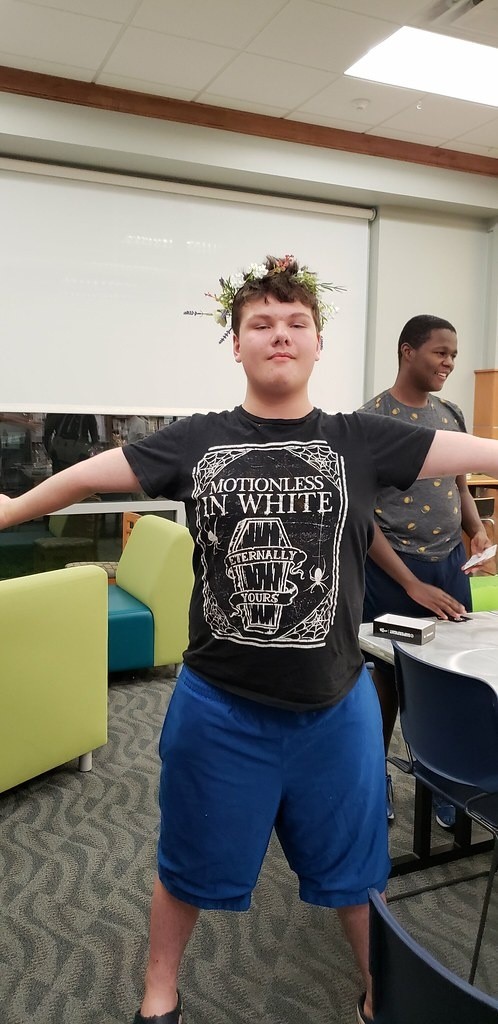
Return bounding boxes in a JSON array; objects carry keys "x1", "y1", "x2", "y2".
[{"x1": 183, "y1": 254, "x2": 348, "y2": 345}]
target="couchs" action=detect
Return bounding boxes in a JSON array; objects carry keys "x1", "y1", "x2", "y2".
[
  {"x1": 108, "y1": 514, "x2": 198, "y2": 680},
  {"x1": 0, "y1": 565, "x2": 107, "y2": 795}
]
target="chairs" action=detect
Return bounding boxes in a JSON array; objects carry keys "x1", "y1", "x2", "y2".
[
  {"x1": 65, "y1": 511, "x2": 143, "y2": 586},
  {"x1": 377, "y1": 639, "x2": 498, "y2": 986},
  {"x1": 466, "y1": 497, "x2": 496, "y2": 546},
  {"x1": 367, "y1": 886, "x2": 498, "y2": 1024},
  {"x1": 35, "y1": 497, "x2": 102, "y2": 574}
]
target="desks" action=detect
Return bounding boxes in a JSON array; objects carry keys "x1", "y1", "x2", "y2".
[
  {"x1": 19, "y1": 465, "x2": 51, "y2": 488},
  {"x1": 465, "y1": 473, "x2": 498, "y2": 498},
  {"x1": 357, "y1": 610, "x2": 498, "y2": 696}
]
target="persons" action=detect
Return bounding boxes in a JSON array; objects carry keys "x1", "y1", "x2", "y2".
[
  {"x1": 120, "y1": 415, "x2": 147, "y2": 445},
  {"x1": 0, "y1": 254, "x2": 498, "y2": 1024},
  {"x1": 42, "y1": 413, "x2": 102, "y2": 477},
  {"x1": 347, "y1": 315, "x2": 498, "y2": 821}
]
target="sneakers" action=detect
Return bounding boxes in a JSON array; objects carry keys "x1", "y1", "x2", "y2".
[
  {"x1": 133, "y1": 986, "x2": 183, "y2": 1024},
  {"x1": 385, "y1": 774, "x2": 395, "y2": 819},
  {"x1": 357, "y1": 990, "x2": 375, "y2": 1023},
  {"x1": 432, "y1": 790, "x2": 455, "y2": 831}
]
